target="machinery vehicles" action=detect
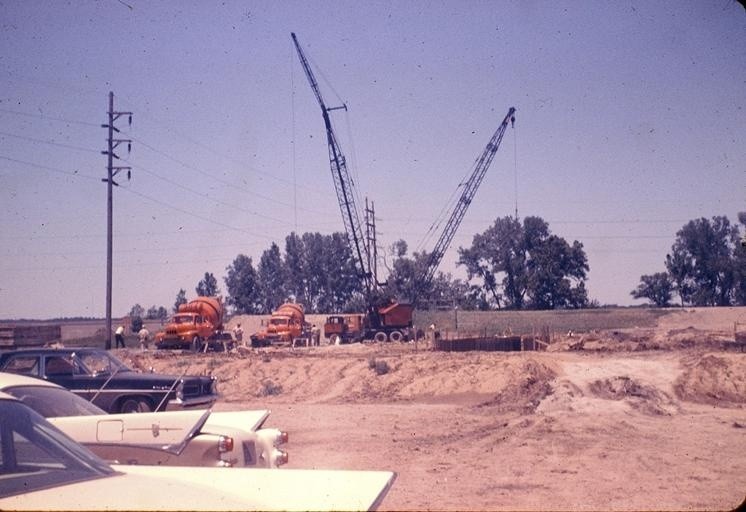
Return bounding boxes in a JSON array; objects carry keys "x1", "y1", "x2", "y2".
[
  {"x1": 262, "y1": 300, "x2": 311, "y2": 347},
  {"x1": 148, "y1": 293, "x2": 230, "y2": 351},
  {"x1": 321, "y1": 302, "x2": 418, "y2": 345}
]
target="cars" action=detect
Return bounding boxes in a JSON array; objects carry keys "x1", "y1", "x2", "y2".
[
  {"x1": 0, "y1": 370, "x2": 289, "y2": 468},
  {"x1": 0, "y1": 346, "x2": 220, "y2": 415},
  {"x1": 1, "y1": 391, "x2": 399, "y2": 512}
]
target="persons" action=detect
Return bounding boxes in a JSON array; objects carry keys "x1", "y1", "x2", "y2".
[
  {"x1": 112, "y1": 324, "x2": 126, "y2": 348},
  {"x1": 310, "y1": 325, "x2": 321, "y2": 346},
  {"x1": 232, "y1": 322, "x2": 244, "y2": 341},
  {"x1": 136, "y1": 324, "x2": 149, "y2": 350}
]
